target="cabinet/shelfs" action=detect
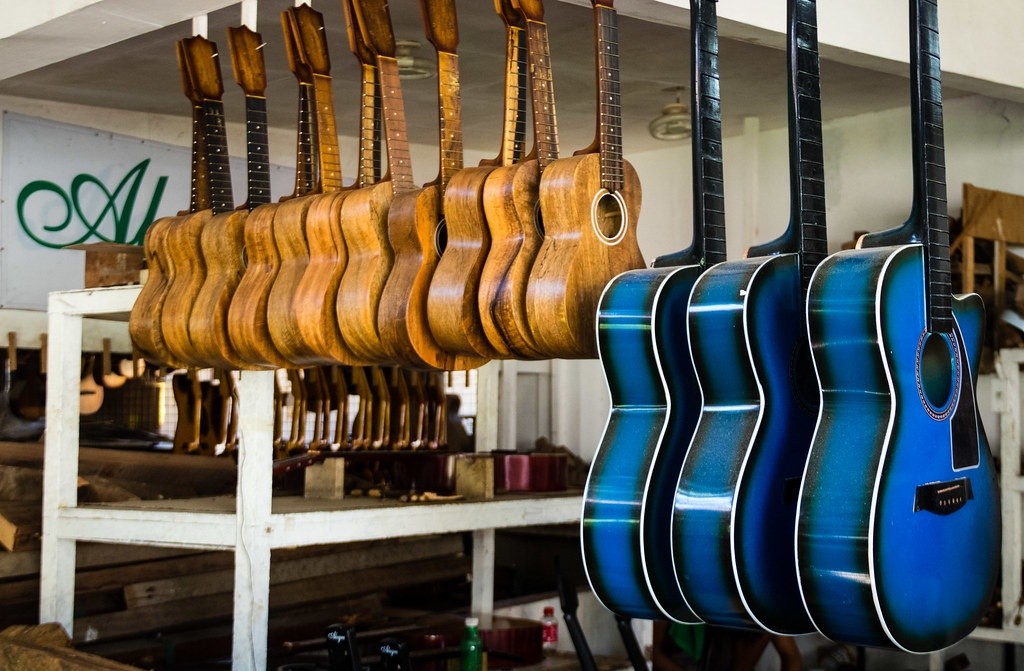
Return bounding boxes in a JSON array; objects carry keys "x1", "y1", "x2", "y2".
[{"x1": 38, "y1": 284, "x2": 585, "y2": 671}]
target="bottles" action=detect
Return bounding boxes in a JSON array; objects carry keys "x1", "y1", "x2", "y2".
[
  {"x1": 539, "y1": 606, "x2": 559, "y2": 651},
  {"x1": 459, "y1": 617, "x2": 483, "y2": 671}
]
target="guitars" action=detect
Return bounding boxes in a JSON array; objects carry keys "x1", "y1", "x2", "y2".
[
  {"x1": 577, "y1": 0, "x2": 731, "y2": 628},
  {"x1": 790, "y1": 0, "x2": 1000, "y2": 657},
  {"x1": 128, "y1": 0, "x2": 562, "y2": 385},
  {"x1": 666, "y1": 0, "x2": 831, "y2": 640},
  {"x1": 525, "y1": 0, "x2": 649, "y2": 361}
]
